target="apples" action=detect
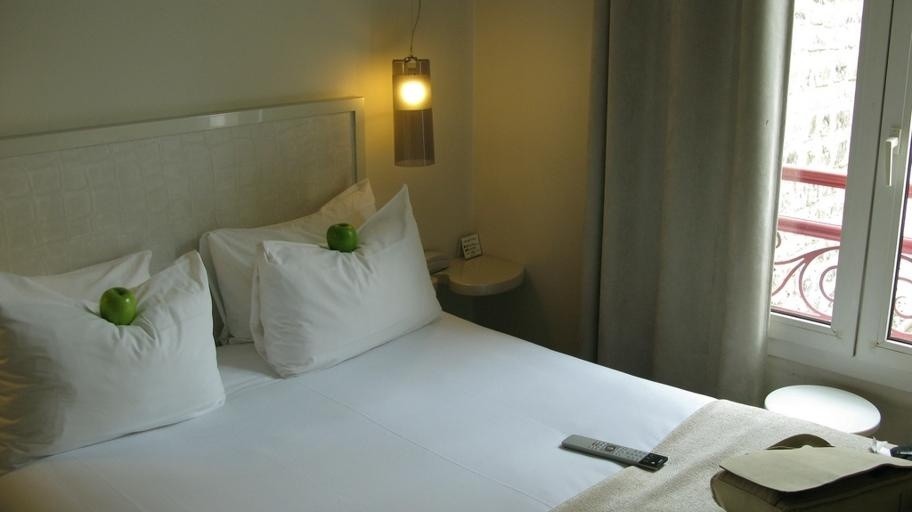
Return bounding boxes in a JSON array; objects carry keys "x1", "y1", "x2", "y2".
[
  {"x1": 326, "y1": 222, "x2": 359, "y2": 252},
  {"x1": 100, "y1": 286, "x2": 138, "y2": 327}
]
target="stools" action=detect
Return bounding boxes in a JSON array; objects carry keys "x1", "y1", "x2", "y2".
[{"x1": 760, "y1": 380, "x2": 882, "y2": 437}]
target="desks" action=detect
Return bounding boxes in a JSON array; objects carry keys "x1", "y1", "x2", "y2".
[{"x1": 428, "y1": 250, "x2": 529, "y2": 324}]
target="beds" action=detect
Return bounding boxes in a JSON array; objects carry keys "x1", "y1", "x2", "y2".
[{"x1": 0, "y1": 89, "x2": 912, "y2": 512}]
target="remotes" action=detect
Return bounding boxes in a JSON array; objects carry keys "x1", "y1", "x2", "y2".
[{"x1": 562, "y1": 433, "x2": 669, "y2": 472}]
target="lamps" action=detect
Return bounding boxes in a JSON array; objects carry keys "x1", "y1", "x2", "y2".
[{"x1": 387, "y1": 1, "x2": 441, "y2": 171}]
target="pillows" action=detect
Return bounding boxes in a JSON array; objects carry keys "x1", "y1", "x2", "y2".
[
  {"x1": 198, "y1": 175, "x2": 381, "y2": 348},
  {"x1": 1, "y1": 246, "x2": 229, "y2": 479},
  {"x1": 247, "y1": 177, "x2": 446, "y2": 381},
  {"x1": 20, "y1": 247, "x2": 156, "y2": 302}
]
